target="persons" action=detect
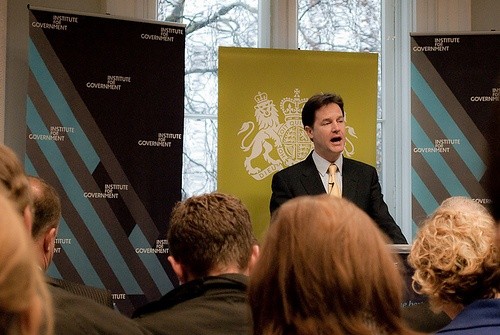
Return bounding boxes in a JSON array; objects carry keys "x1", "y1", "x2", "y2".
[
  {"x1": 269, "y1": 93, "x2": 409, "y2": 245},
  {"x1": 0, "y1": 143, "x2": 500, "y2": 335}
]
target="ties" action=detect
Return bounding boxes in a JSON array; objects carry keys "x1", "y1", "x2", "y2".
[{"x1": 327, "y1": 164, "x2": 341, "y2": 199}]
